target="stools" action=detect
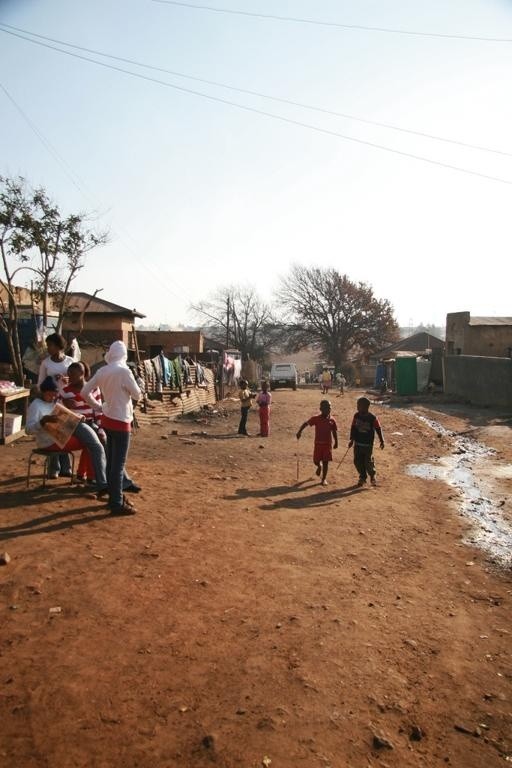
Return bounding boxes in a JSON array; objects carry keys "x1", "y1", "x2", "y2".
[{"x1": 27, "y1": 447, "x2": 75, "y2": 491}]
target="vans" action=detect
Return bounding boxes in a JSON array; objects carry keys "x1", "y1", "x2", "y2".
[{"x1": 269, "y1": 363, "x2": 297, "y2": 390}]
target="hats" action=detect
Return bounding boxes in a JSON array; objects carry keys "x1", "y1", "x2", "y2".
[{"x1": 40, "y1": 376, "x2": 58, "y2": 391}]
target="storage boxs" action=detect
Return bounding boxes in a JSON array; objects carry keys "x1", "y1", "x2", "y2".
[{"x1": 0, "y1": 413, "x2": 24, "y2": 439}]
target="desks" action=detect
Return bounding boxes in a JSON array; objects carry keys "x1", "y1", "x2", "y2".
[{"x1": 0, "y1": 388, "x2": 31, "y2": 445}]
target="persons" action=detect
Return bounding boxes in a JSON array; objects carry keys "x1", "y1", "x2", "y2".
[
  {"x1": 29, "y1": 333, "x2": 149, "y2": 515},
  {"x1": 238, "y1": 379, "x2": 256, "y2": 437},
  {"x1": 356, "y1": 375, "x2": 360, "y2": 388},
  {"x1": 257, "y1": 381, "x2": 272, "y2": 437},
  {"x1": 349, "y1": 397, "x2": 385, "y2": 487},
  {"x1": 298, "y1": 367, "x2": 346, "y2": 394},
  {"x1": 296, "y1": 399, "x2": 338, "y2": 486},
  {"x1": 379, "y1": 376, "x2": 387, "y2": 393}
]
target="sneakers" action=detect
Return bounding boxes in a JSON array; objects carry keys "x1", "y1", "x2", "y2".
[{"x1": 47, "y1": 468, "x2": 142, "y2": 515}]
